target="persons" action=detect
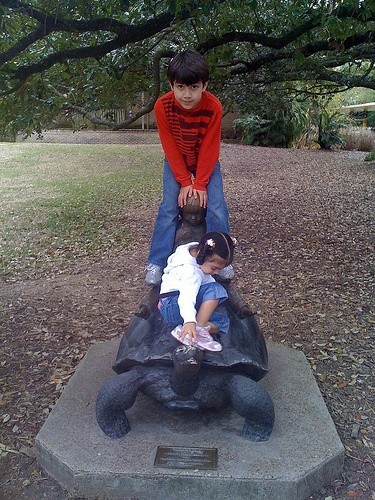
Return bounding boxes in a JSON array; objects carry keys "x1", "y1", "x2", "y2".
[
  {"x1": 145, "y1": 51, "x2": 234, "y2": 288},
  {"x1": 158, "y1": 231, "x2": 242, "y2": 350}
]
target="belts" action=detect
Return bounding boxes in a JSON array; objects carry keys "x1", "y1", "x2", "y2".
[{"x1": 157, "y1": 297, "x2": 168, "y2": 309}]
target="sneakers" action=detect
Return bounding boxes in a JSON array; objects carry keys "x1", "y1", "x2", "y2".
[
  {"x1": 218, "y1": 264, "x2": 234, "y2": 280},
  {"x1": 145, "y1": 263, "x2": 162, "y2": 285},
  {"x1": 196, "y1": 326, "x2": 223, "y2": 352},
  {"x1": 171, "y1": 325, "x2": 196, "y2": 346}
]
